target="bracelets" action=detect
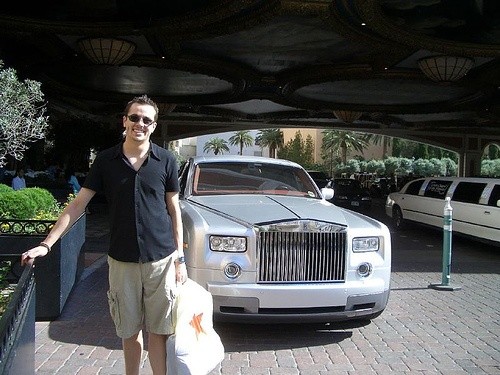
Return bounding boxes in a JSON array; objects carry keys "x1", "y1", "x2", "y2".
[{"x1": 40, "y1": 242, "x2": 51, "y2": 252}]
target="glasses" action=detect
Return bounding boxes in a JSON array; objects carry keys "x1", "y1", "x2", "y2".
[{"x1": 125, "y1": 114, "x2": 156, "y2": 124}]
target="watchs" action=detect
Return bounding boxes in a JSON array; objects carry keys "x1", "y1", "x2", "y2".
[{"x1": 176, "y1": 256, "x2": 184, "y2": 263}]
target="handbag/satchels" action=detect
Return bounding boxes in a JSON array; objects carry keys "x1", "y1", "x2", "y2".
[{"x1": 166, "y1": 279, "x2": 226, "y2": 375}]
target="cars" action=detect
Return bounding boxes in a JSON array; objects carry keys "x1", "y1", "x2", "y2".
[
  {"x1": 322, "y1": 177, "x2": 373, "y2": 214},
  {"x1": 306, "y1": 170, "x2": 329, "y2": 188},
  {"x1": 369, "y1": 178, "x2": 393, "y2": 199},
  {"x1": 176, "y1": 155, "x2": 392, "y2": 326},
  {"x1": 386, "y1": 177, "x2": 499, "y2": 247}
]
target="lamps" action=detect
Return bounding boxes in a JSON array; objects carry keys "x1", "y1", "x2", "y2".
[
  {"x1": 333, "y1": 110, "x2": 363, "y2": 124},
  {"x1": 417, "y1": 53, "x2": 475, "y2": 85},
  {"x1": 76, "y1": 35, "x2": 137, "y2": 67}
]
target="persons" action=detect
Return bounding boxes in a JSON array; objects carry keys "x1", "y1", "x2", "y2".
[
  {"x1": 12, "y1": 169, "x2": 25, "y2": 190},
  {"x1": 21, "y1": 95, "x2": 187, "y2": 375},
  {"x1": 47, "y1": 158, "x2": 90, "y2": 216}
]
